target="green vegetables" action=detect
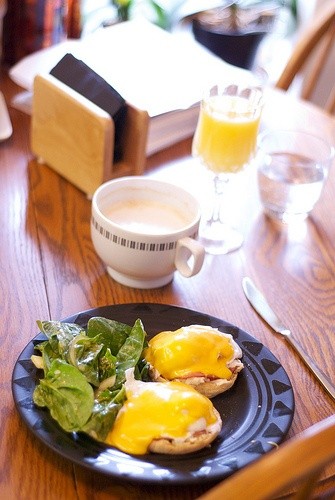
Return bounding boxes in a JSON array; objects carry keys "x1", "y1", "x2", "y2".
[{"x1": 33, "y1": 316, "x2": 154, "y2": 442}]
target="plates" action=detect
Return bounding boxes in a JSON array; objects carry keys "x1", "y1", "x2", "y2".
[{"x1": 11, "y1": 303, "x2": 294, "y2": 485}]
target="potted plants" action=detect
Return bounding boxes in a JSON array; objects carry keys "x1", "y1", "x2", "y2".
[{"x1": 191, "y1": 1, "x2": 274, "y2": 70}]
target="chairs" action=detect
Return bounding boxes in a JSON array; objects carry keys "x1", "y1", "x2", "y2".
[{"x1": 274, "y1": 10, "x2": 335, "y2": 115}]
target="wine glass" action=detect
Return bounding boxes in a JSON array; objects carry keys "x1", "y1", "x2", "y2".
[{"x1": 190, "y1": 80, "x2": 267, "y2": 254}]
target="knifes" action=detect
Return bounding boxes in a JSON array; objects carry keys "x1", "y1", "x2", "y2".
[{"x1": 242, "y1": 276, "x2": 335, "y2": 402}]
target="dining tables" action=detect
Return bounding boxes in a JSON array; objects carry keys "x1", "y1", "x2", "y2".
[{"x1": 1, "y1": 69, "x2": 335, "y2": 500}]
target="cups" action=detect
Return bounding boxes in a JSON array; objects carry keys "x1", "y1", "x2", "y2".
[
  {"x1": 90, "y1": 175, "x2": 205, "y2": 289},
  {"x1": 257, "y1": 129, "x2": 335, "y2": 223}
]
